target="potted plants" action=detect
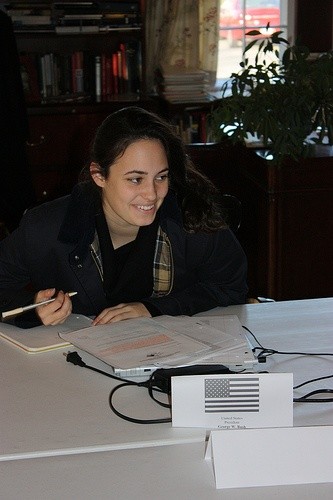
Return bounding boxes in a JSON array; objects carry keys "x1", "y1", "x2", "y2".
[{"x1": 206, "y1": 23, "x2": 333, "y2": 178}]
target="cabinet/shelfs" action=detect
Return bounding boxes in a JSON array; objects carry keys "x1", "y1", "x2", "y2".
[{"x1": 0, "y1": 0, "x2": 152, "y2": 203}]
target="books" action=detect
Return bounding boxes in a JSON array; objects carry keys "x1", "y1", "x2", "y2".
[
  {"x1": 171, "y1": 112, "x2": 207, "y2": 142},
  {"x1": 19, "y1": 43, "x2": 129, "y2": 97},
  {"x1": 157, "y1": 64, "x2": 209, "y2": 103}
]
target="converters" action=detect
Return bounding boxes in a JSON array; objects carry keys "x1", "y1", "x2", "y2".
[{"x1": 153, "y1": 363, "x2": 229, "y2": 393}]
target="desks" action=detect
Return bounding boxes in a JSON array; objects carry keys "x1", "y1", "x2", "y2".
[
  {"x1": 219, "y1": 119, "x2": 333, "y2": 299},
  {"x1": 0, "y1": 295, "x2": 333, "y2": 500}
]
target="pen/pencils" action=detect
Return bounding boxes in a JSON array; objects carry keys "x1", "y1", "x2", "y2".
[{"x1": 2, "y1": 292, "x2": 78, "y2": 316}]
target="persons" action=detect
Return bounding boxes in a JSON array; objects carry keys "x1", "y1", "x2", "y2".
[{"x1": 0, "y1": 107, "x2": 249, "y2": 330}]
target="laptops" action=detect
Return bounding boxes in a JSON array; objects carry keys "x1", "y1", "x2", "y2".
[{"x1": 114, "y1": 315, "x2": 258, "y2": 377}]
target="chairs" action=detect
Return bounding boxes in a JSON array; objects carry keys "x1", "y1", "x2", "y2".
[{"x1": 185, "y1": 142, "x2": 279, "y2": 302}]
